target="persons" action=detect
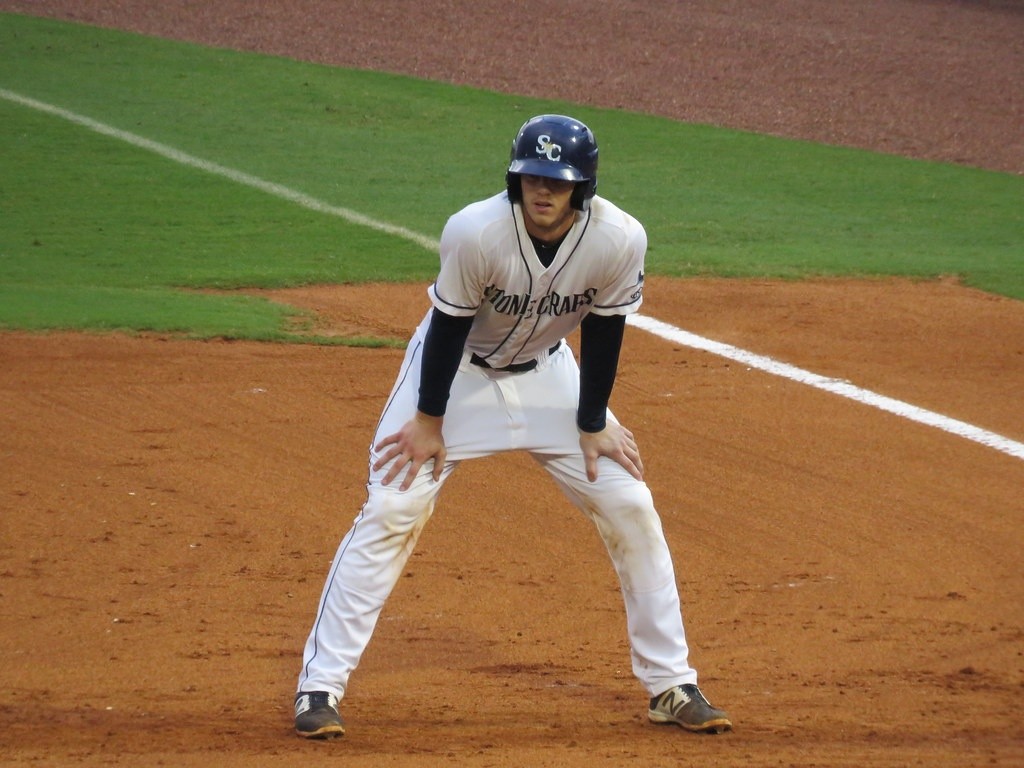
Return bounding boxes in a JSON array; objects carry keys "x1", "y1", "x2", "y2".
[{"x1": 295, "y1": 113, "x2": 733, "y2": 734}]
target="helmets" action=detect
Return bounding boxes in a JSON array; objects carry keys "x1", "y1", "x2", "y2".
[{"x1": 505, "y1": 114, "x2": 598, "y2": 212}]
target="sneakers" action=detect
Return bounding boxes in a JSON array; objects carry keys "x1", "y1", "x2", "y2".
[
  {"x1": 292, "y1": 690, "x2": 346, "y2": 740},
  {"x1": 647, "y1": 684, "x2": 733, "y2": 734}
]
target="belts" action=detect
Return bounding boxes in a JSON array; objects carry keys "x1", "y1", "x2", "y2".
[{"x1": 470, "y1": 340, "x2": 561, "y2": 372}]
